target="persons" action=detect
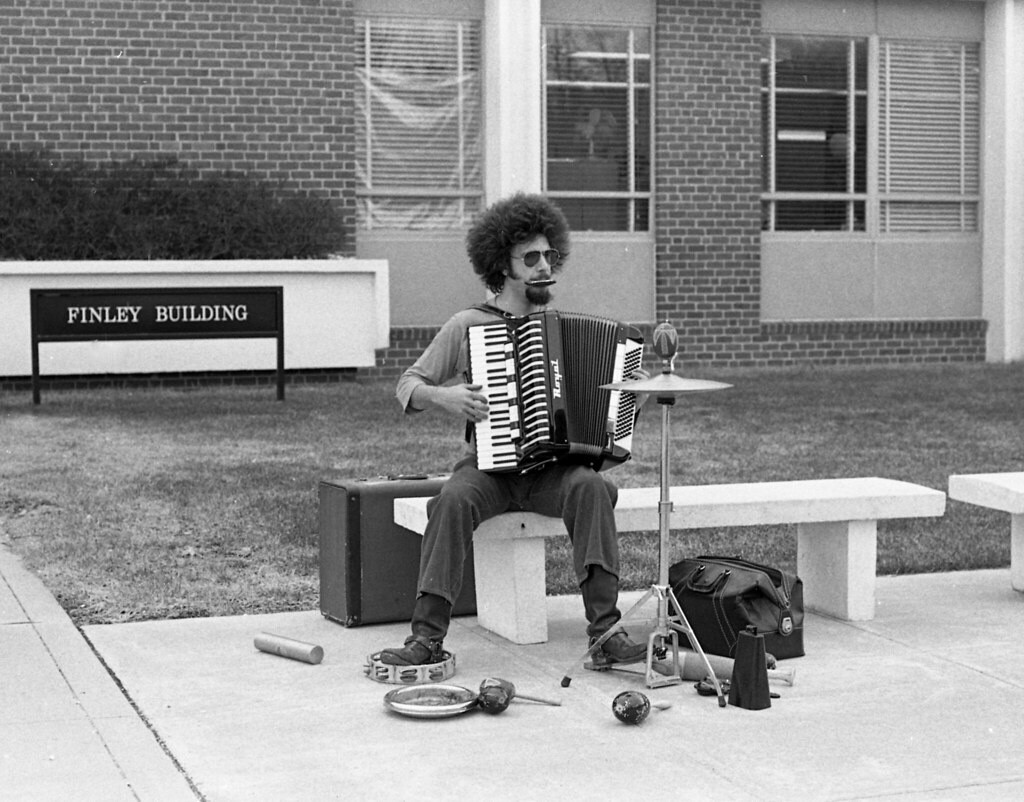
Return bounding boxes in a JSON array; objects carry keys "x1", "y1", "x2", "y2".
[{"x1": 381, "y1": 191, "x2": 657, "y2": 669}]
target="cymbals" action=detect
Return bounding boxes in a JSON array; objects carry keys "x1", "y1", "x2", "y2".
[{"x1": 362, "y1": 647, "x2": 456, "y2": 685}]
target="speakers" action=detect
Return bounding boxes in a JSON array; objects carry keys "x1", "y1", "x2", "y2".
[{"x1": 320, "y1": 473, "x2": 477, "y2": 629}]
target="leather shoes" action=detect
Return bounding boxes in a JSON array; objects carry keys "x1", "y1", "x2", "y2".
[
  {"x1": 381, "y1": 635, "x2": 443, "y2": 665},
  {"x1": 588, "y1": 632, "x2": 658, "y2": 666}
]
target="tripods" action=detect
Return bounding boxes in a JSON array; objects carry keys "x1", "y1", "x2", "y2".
[{"x1": 561, "y1": 403, "x2": 728, "y2": 706}]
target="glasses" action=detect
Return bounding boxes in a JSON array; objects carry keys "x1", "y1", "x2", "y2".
[{"x1": 509, "y1": 249, "x2": 562, "y2": 268}]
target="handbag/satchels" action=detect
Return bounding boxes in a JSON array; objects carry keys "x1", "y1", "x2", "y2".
[{"x1": 668, "y1": 555, "x2": 806, "y2": 660}]
